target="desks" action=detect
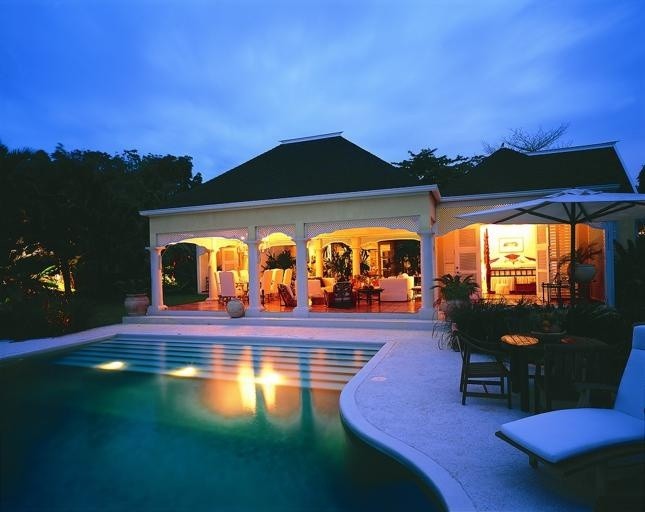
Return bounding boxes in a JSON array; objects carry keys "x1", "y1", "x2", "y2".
[
  {"x1": 357, "y1": 290, "x2": 384, "y2": 306},
  {"x1": 542, "y1": 280, "x2": 598, "y2": 306}
]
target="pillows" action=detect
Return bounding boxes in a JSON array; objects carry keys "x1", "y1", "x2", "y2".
[
  {"x1": 308, "y1": 277, "x2": 325, "y2": 287},
  {"x1": 396, "y1": 272, "x2": 408, "y2": 278}
]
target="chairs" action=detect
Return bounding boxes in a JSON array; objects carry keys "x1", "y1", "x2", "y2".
[
  {"x1": 379, "y1": 276, "x2": 414, "y2": 303},
  {"x1": 214, "y1": 268, "x2": 292, "y2": 307},
  {"x1": 308, "y1": 277, "x2": 336, "y2": 305},
  {"x1": 451, "y1": 317, "x2": 644, "y2": 510}
]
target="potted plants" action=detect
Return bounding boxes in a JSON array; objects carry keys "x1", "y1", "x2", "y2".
[
  {"x1": 113, "y1": 277, "x2": 151, "y2": 315},
  {"x1": 429, "y1": 272, "x2": 481, "y2": 322},
  {"x1": 566, "y1": 240, "x2": 605, "y2": 286},
  {"x1": 323, "y1": 243, "x2": 354, "y2": 309}
]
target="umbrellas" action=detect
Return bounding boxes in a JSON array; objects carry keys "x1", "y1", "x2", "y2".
[{"x1": 455, "y1": 188, "x2": 645, "y2": 335}]
